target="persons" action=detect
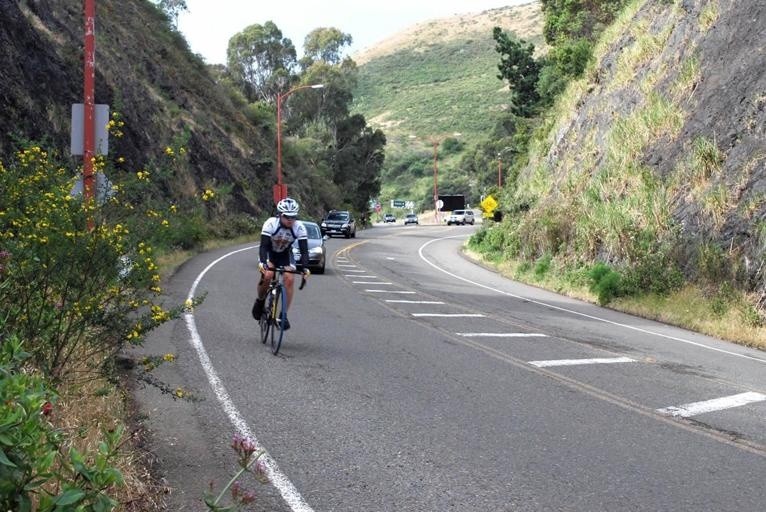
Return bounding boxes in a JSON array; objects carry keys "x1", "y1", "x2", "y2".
[{"x1": 250, "y1": 197, "x2": 310, "y2": 331}]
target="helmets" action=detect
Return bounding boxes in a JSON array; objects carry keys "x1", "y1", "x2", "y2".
[{"x1": 276, "y1": 197, "x2": 300, "y2": 217}]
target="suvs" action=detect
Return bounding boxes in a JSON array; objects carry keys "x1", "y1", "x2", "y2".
[
  {"x1": 320, "y1": 209, "x2": 357, "y2": 239},
  {"x1": 404, "y1": 213, "x2": 418, "y2": 225},
  {"x1": 383, "y1": 214, "x2": 396, "y2": 223}
]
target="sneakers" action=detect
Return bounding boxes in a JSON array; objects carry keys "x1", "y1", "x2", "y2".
[
  {"x1": 278, "y1": 312, "x2": 291, "y2": 330},
  {"x1": 251, "y1": 297, "x2": 265, "y2": 320}
]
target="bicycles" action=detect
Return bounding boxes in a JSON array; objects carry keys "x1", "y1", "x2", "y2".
[{"x1": 258, "y1": 263, "x2": 308, "y2": 356}]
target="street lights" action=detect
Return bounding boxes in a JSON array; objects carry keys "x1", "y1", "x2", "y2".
[
  {"x1": 273, "y1": 83, "x2": 325, "y2": 206},
  {"x1": 497, "y1": 146, "x2": 514, "y2": 191},
  {"x1": 433, "y1": 132, "x2": 462, "y2": 223}
]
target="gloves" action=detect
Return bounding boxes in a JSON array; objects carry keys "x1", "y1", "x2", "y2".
[
  {"x1": 257, "y1": 258, "x2": 269, "y2": 275},
  {"x1": 301, "y1": 268, "x2": 311, "y2": 280}
]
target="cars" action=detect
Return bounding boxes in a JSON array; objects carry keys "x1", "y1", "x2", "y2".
[
  {"x1": 447, "y1": 210, "x2": 475, "y2": 226},
  {"x1": 291, "y1": 219, "x2": 328, "y2": 275}
]
